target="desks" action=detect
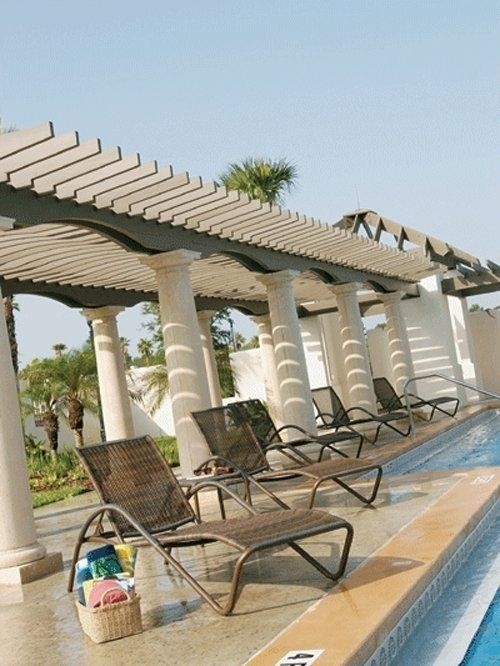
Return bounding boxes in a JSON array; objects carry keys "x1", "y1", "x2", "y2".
[{"x1": 175, "y1": 469, "x2": 245, "y2": 521}]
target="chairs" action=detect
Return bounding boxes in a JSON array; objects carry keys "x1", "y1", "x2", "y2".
[
  {"x1": 310, "y1": 385, "x2": 410, "y2": 443},
  {"x1": 373, "y1": 377, "x2": 459, "y2": 422},
  {"x1": 231, "y1": 400, "x2": 362, "y2": 465},
  {"x1": 189, "y1": 404, "x2": 382, "y2": 511},
  {"x1": 66, "y1": 435, "x2": 354, "y2": 616}
]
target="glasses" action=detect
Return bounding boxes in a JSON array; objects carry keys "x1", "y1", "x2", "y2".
[{"x1": 194, "y1": 468, "x2": 211, "y2": 476}]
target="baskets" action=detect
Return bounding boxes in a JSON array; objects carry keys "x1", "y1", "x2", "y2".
[{"x1": 75, "y1": 588, "x2": 142, "y2": 644}]
